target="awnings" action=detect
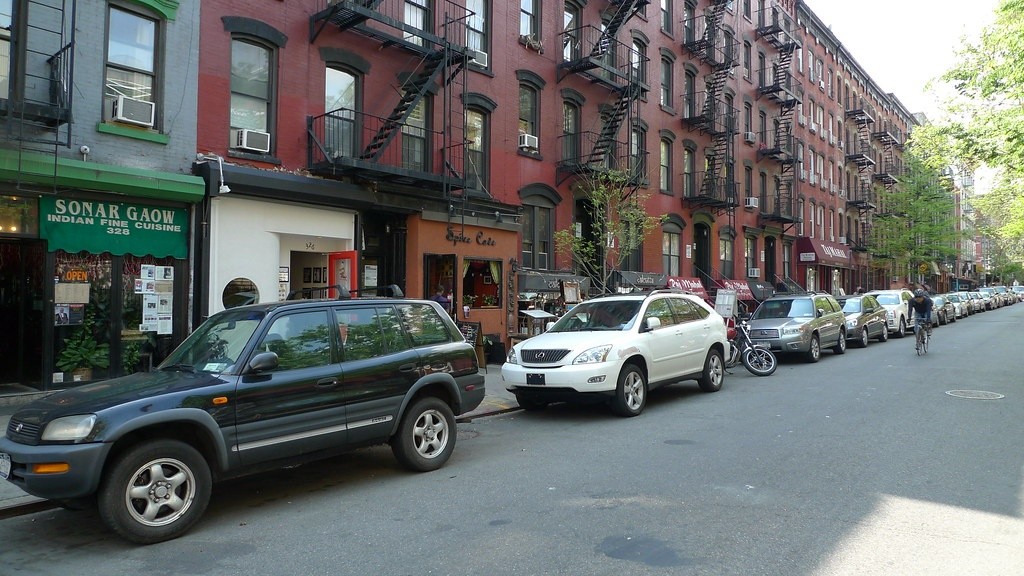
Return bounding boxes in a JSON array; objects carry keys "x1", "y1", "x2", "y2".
[
  {"x1": 796, "y1": 236, "x2": 857, "y2": 270},
  {"x1": 665, "y1": 275, "x2": 708, "y2": 300},
  {"x1": 746, "y1": 281, "x2": 776, "y2": 300},
  {"x1": 929, "y1": 260, "x2": 941, "y2": 276},
  {"x1": 714, "y1": 279, "x2": 753, "y2": 301}
]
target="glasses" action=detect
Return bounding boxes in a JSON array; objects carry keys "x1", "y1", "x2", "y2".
[{"x1": 915, "y1": 295, "x2": 922, "y2": 298}]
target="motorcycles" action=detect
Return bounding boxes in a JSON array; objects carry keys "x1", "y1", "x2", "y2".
[{"x1": 722, "y1": 304, "x2": 778, "y2": 376}]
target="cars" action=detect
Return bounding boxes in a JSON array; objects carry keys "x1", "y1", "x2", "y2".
[
  {"x1": 976, "y1": 286, "x2": 1024, "y2": 309},
  {"x1": 929, "y1": 295, "x2": 956, "y2": 329},
  {"x1": 834, "y1": 295, "x2": 888, "y2": 348},
  {"x1": 954, "y1": 292, "x2": 975, "y2": 315},
  {"x1": 945, "y1": 294, "x2": 968, "y2": 319},
  {"x1": 969, "y1": 292, "x2": 995, "y2": 313}
]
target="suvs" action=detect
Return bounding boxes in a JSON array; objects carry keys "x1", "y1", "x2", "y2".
[
  {"x1": 863, "y1": 288, "x2": 917, "y2": 338},
  {"x1": 747, "y1": 292, "x2": 848, "y2": 362},
  {"x1": 500, "y1": 289, "x2": 731, "y2": 418},
  {"x1": 0, "y1": 300, "x2": 486, "y2": 543}
]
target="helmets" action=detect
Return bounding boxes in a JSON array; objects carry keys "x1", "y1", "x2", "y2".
[{"x1": 914, "y1": 289, "x2": 924, "y2": 297}]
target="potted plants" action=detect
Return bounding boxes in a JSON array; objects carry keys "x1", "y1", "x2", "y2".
[{"x1": 56, "y1": 334, "x2": 110, "y2": 383}]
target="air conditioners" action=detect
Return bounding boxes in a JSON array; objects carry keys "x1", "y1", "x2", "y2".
[
  {"x1": 893, "y1": 276, "x2": 899, "y2": 281},
  {"x1": 517, "y1": 134, "x2": 538, "y2": 149},
  {"x1": 838, "y1": 237, "x2": 846, "y2": 244},
  {"x1": 748, "y1": 268, "x2": 760, "y2": 278},
  {"x1": 468, "y1": 49, "x2": 487, "y2": 69},
  {"x1": 743, "y1": 132, "x2": 755, "y2": 144},
  {"x1": 110, "y1": 94, "x2": 155, "y2": 128},
  {"x1": 744, "y1": 197, "x2": 758, "y2": 208},
  {"x1": 798, "y1": 81, "x2": 845, "y2": 197},
  {"x1": 238, "y1": 129, "x2": 271, "y2": 153},
  {"x1": 743, "y1": 68, "x2": 749, "y2": 77}
]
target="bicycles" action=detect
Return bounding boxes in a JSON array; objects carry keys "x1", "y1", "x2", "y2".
[{"x1": 907, "y1": 318, "x2": 930, "y2": 356}]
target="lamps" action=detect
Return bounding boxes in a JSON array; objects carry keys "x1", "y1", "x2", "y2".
[{"x1": 196, "y1": 153, "x2": 231, "y2": 194}]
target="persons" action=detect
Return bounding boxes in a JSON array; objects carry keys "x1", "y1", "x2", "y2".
[
  {"x1": 428, "y1": 286, "x2": 449, "y2": 314},
  {"x1": 907, "y1": 288, "x2": 932, "y2": 350}
]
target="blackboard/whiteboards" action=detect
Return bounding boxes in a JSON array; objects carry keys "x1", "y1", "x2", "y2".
[{"x1": 455, "y1": 320, "x2": 487, "y2": 368}]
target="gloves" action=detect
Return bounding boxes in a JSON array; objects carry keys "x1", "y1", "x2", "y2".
[
  {"x1": 925, "y1": 318, "x2": 932, "y2": 324},
  {"x1": 907, "y1": 317, "x2": 911, "y2": 325}
]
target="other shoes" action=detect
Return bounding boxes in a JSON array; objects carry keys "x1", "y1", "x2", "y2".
[
  {"x1": 915, "y1": 340, "x2": 922, "y2": 350},
  {"x1": 927, "y1": 328, "x2": 932, "y2": 336}
]
